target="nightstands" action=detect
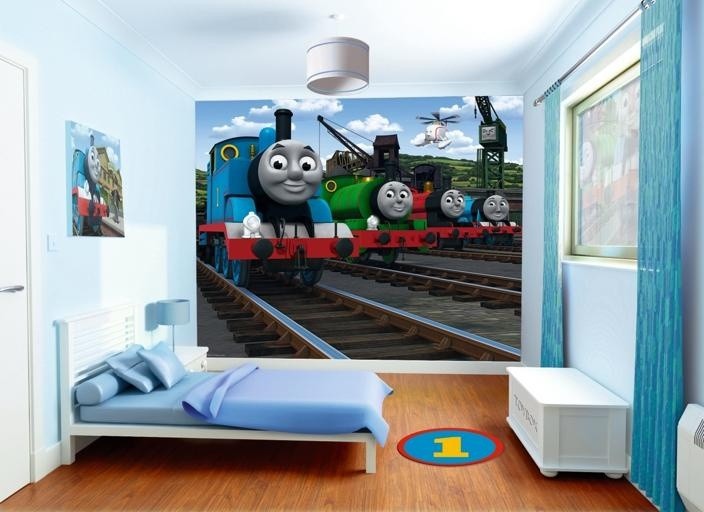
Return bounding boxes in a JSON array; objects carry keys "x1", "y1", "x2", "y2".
[{"x1": 170, "y1": 346, "x2": 209, "y2": 373}]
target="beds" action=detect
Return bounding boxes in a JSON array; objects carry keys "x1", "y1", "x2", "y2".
[
  {"x1": 54, "y1": 302, "x2": 377, "y2": 474},
  {"x1": 76, "y1": 340, "x2": 186, "y2": 407}
]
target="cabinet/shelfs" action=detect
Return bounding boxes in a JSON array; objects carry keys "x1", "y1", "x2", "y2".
[{"x1": 505, "y1": 365, "x2": 630, "y2": 480}]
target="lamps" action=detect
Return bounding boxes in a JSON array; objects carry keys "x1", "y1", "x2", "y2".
[
  {"x1": 156, "y1": 298, "x2": 190, "y2": 354},
  {"x1": 306, "y1": 36, "x2": 370, "y2": 96}
]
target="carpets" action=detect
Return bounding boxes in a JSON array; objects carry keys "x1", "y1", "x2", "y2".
[{"x1": 396, "y1": 427, "x2": 506, "y2": 467}]
